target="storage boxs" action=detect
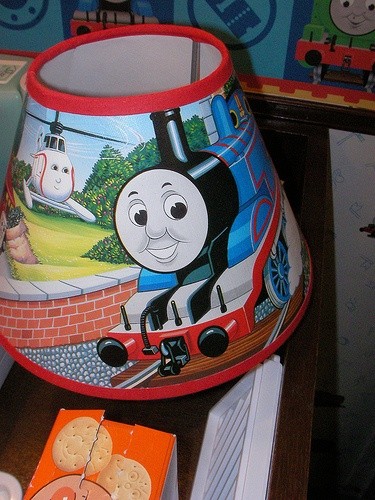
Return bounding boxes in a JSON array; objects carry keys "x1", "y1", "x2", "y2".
[{"x1": 21, "y1": 409, "x2": 177, "y2": 500}]
